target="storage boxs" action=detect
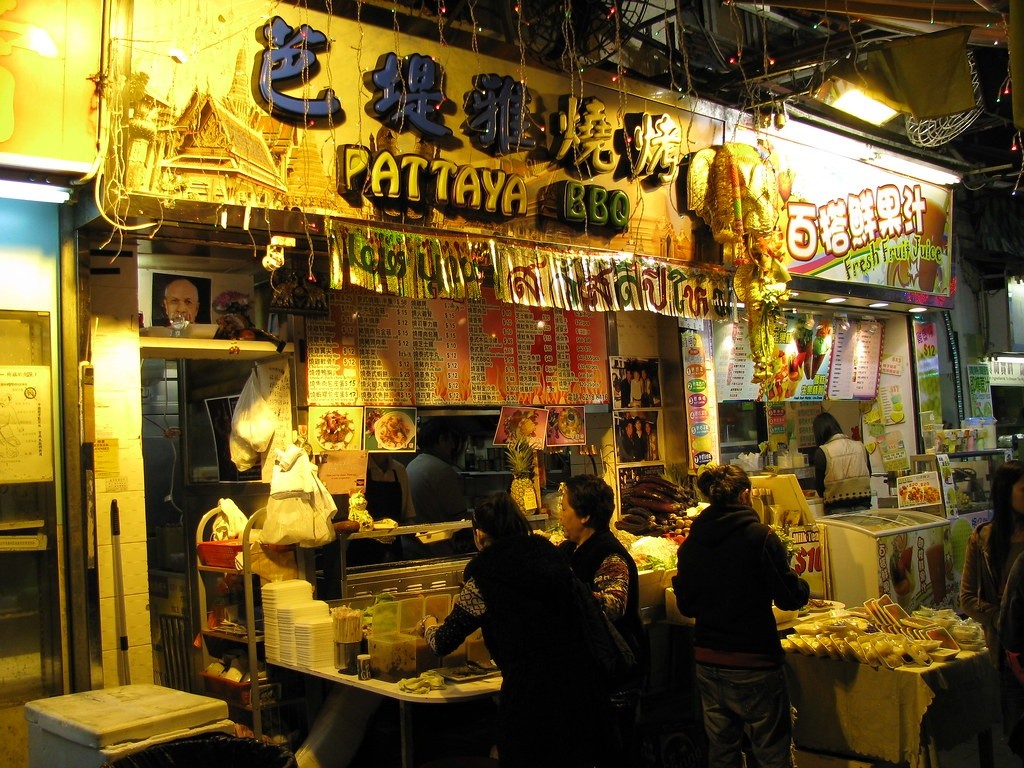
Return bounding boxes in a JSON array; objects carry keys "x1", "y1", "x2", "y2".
[
  {"x1": 366, "y1": 593, "x2": 495, "y2": 683},
  {"x1": 199, "y1": 539, "x2": 242, "y2": 569},
  {"x1": 199, "y1": 672, "x2": 267, "y2": 706},
  {"x1": 617, "y1": 529, "x2": 696, "y2": 626},
  {"x1": 781, "y1": 594, "x2": 984, "y2": 669}
]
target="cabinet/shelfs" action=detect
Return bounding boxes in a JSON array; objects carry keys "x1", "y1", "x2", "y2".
[{"x1": 195, "y1": 504, "x2": 316, "y2": 742}]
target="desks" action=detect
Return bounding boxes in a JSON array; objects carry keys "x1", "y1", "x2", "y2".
[
  {"x1": 782, "y1": 644, "x2": 995, "y2": 768},
  {"x1": 267, "y1": 657, "x2": 502, "y2": 768}
]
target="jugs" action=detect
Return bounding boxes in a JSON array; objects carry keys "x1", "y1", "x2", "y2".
[{"x1": 962, "y1": 416, "x2": 998, "y2": 449}]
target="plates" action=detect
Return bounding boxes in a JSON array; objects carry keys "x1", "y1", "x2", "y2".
[
  {"x1": 260, "y1": 579, "x2": 335, "y2": 668},
  {"x1": 374, "y1": 411, "x2": 415, "y2": 450},
  {"x1": 315, "y1": 410, "x2": 355, "y2": 451},
  {"x1": 809, "y1": 599, "x2": 846, "y2": 612},
  {"x1": 429, "y1": 665, "x2": 501, "y2": 681},
  {"x1": 558, "y1": 407, "x2": 582, "y2": 439}
]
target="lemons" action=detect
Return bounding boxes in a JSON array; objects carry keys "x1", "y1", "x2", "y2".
[{"x1": 891, "y1": 413, "x2": 904, "y2": 422}]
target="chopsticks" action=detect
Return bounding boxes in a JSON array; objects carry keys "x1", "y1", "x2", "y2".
[{"x1": 330, "y1": 605, "x2": 363, "y2": 642}]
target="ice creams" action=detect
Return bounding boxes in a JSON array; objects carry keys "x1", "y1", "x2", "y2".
[
  {"x1": 811, "y1": 338, "x2": 829, "y2": 379},
  {"x1": 792, "y1": 324, "x2": 813, "y2": 379}
]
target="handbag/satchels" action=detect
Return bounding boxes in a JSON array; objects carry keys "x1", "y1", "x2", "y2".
[
  {"x1": 258, "y1": 494, "x2": 315, "y2": 546},
  {"x1": 566, "y1": 562, "x2": 635, "y2": 678},
  {"x1": 231, "y1": 367, "x2": 277, "y2": 452},
  {"x1": 611, "y1": 606, "x2": 649, "y2": 672},
  {"x1": 297, "y1": 490, "x2": 336, "y2": 548},
  {"x1": 228, "y1": 430, "x2": 259, "y2": 472}
]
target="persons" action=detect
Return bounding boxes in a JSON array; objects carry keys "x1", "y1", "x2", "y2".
[
  {"x1": 554, "y1": 474, "x2": 642, "y2": 656},
  {"x1": 961, "y1": 459, "x2": 1024, "y2": 756},
  {"x1": 346, "y1": 453, "x2": 417, "y2": 568},
  {"x1": 162, "y1": 279, "x2": 200, "y2": 326},
  {"x1": 813, "y1": 413, "x2": 872, "y2": 516},
  {"x1": 672, "y1": 466, "x2": 810, "y2": 768},
  {"x1": 613, "y1": 367, "x2": 658, "y2": 461},
  {"x1": 404, "y1": 419, "x2": 475, "y2": 561},
  {"x1": 411, "y1": 490, "x2": 639, "y2": 768}
]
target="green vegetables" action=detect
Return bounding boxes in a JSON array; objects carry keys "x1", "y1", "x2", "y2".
[{"x1": 635, "y1": 536, "x2": 676, "y2": 569}]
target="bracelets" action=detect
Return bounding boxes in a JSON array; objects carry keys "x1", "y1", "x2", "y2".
[{"x1": 422, "y1": 615, "x2": 439, "y2": 627}]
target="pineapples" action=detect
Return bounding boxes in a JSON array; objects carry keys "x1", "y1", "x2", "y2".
[{"x1": 505, "y1": 438, "x2": 539, "y2": 516}]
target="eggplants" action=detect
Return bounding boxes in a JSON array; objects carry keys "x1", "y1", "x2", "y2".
[{"x1": 616, "y1": 475, "x2": 695, "y2": 534}]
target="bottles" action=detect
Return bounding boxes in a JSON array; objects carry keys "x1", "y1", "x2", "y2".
[{"x1": 752, "y1": 488, "x2": 774, "y2": 525}]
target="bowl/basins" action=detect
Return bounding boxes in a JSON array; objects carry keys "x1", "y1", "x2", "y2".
[
  {"x1": 205, "y1": 651, "x2": 266, "y2": 683},
  {"x1": 414, "y1": 527, "x2": 454, "y2": 544},
  {"x1": 748, "y1": 430, "x2": 757, "y2": 440}
]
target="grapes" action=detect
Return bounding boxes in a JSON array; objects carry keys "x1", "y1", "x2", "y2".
[{"x1": 957, "y1": 493, "x2": 969, "y2": 505}]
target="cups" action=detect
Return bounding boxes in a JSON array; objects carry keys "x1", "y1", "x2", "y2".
[
  {"x1": 778, "y1": 455, "x2": 793, "y2": 468},
  {"x1": 333, "y1": 641, "x2": 361, "y2": 669},
  {"x1": 943, "y1": 428, "x2": 984, "y2": 453},
  {"x1": 919, "y1": 198, "x2": 945, "y2": 293},
  {"x1": 919, "y1": 410, "x2": 944, "y2": 455},
  {"x1": 792, "y1": 456, "x2": 804, "y2": 468}
]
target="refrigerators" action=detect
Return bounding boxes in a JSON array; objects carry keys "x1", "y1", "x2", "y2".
[{"x1": 815, "y1": 507, "x2": 952, "y2": 618}]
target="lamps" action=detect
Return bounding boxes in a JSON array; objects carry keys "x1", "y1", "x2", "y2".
[{"x1": 773, "y1": 100, "x2": 785, "y2": 131}]
